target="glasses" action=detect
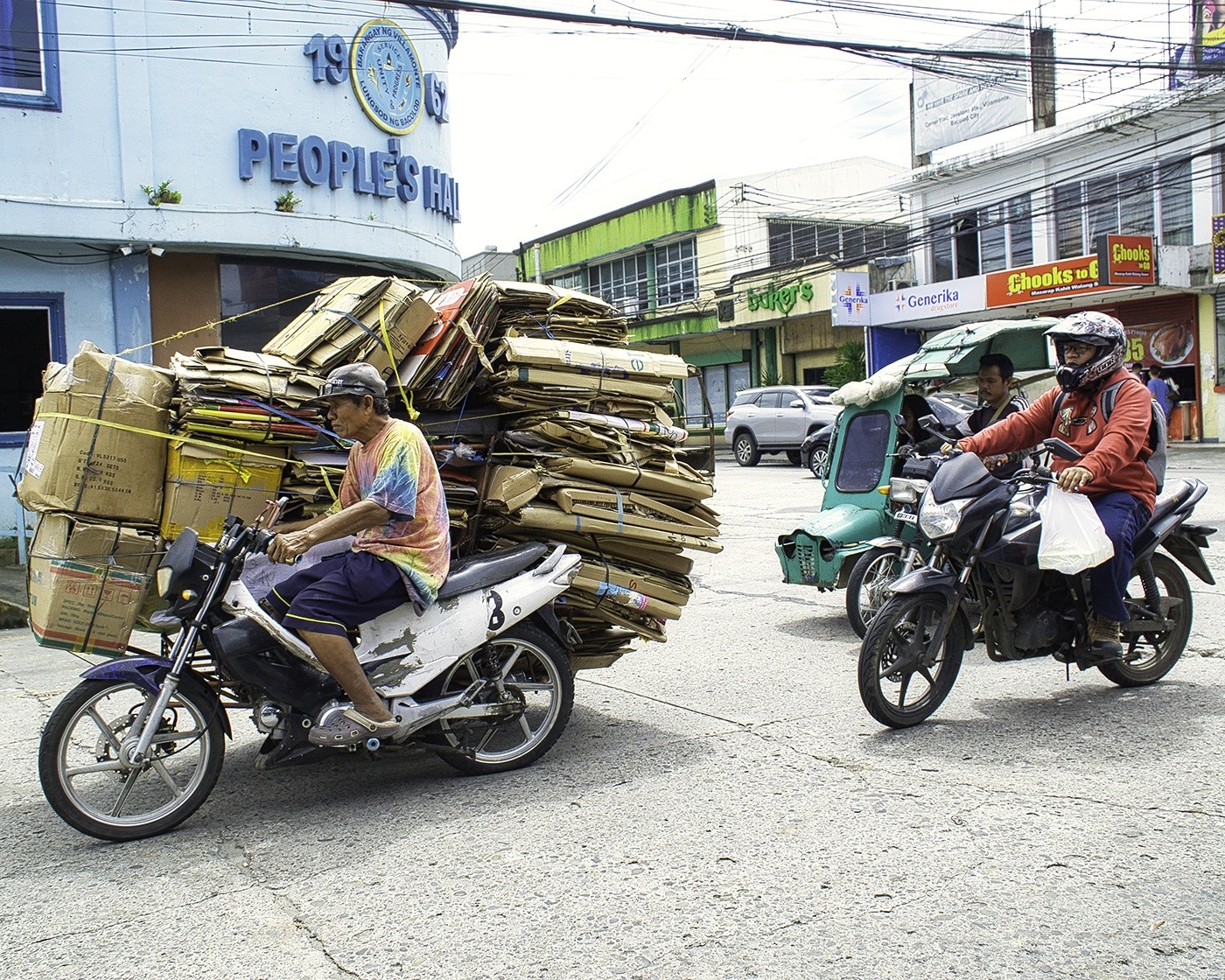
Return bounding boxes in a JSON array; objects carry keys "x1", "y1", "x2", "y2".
[
  {"x1": 319, "y1": 383, "x2": 376, "y2": 395},
  {"x1": 1057, "y1": 343, "x2": 1090, "y2": 355}
]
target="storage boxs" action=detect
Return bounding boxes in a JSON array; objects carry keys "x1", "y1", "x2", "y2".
[
  {"x1": 20, "y1": 339, "x2": 181, "y2": 526},
  {"x1": 161, "y1": 431, "x2": 288, "y2": 545},
  {"x1": 26, "y1": 510, "x2": 166, "y2": 655}
]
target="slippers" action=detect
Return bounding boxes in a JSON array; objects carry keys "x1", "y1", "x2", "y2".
[{"x1": 308, "y1": 708, "x2": 401, "y2": 747}]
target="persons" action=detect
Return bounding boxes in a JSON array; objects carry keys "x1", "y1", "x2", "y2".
[
  {"x1": 939, "y1": 312, "x2": 1153, "y2": 663},
  {"x1": 1146, "y1": 365, "x2": 1172, "y2": 428},
  {"x1": 1129, "y1": 362, "x2": 1144, "y2": 383},
  {"x1": 898, "y1": 353, "x2": 1032, "y2": 483},
  {"x1": 257, "y1": 361, "x2": 451, "y2": 751},
  {"x1": 892, "y1": 393, "x2": 946, "y2": 479}
]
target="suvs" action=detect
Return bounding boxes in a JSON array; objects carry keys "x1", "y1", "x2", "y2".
[{"x1": 725, "y1": 384, "x2": 846, "y2": 467}]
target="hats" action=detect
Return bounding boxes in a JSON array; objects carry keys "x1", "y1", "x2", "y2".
[{"x1": 322, "y1": 363, "x2": 388, "y2": 397}]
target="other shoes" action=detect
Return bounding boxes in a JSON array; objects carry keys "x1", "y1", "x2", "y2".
[{"x1": 973, "y1": 617, "x2": 985, "y2": 642}]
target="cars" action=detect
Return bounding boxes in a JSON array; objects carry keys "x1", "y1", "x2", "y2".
[{"x1": 799, "y1": 390, "x2": 982, "y2": 479}]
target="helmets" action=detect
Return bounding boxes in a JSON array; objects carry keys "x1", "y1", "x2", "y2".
[{"x1": 1041, "y1": 311, "x2": 1128, "y2": 393}]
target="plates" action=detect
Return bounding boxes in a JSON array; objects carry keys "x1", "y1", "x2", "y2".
[{"x1": 1149, "y1": 324, "x2": 1195, "y2": 365}]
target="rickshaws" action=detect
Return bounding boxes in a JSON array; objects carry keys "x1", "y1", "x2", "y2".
[
  {"x1": 778, "y1": 323, "x2": 1220, "y2": 728},
  {"x1": 38, "y1": 494, "x2": 586, "y2": 843}
]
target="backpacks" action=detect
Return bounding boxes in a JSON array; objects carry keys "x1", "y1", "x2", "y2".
[{"x1": 1052, "y1": 391, "x2": 1167, "y2": 495}]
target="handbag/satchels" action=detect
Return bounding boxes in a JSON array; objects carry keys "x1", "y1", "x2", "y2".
[{"x1": 1036, "y1": 472, "x2": 1115, "y2": 574}]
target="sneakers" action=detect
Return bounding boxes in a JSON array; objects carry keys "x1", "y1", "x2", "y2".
[{"x1": 1087, "y1": 612, "x2": 1122, "y2": 656}]
